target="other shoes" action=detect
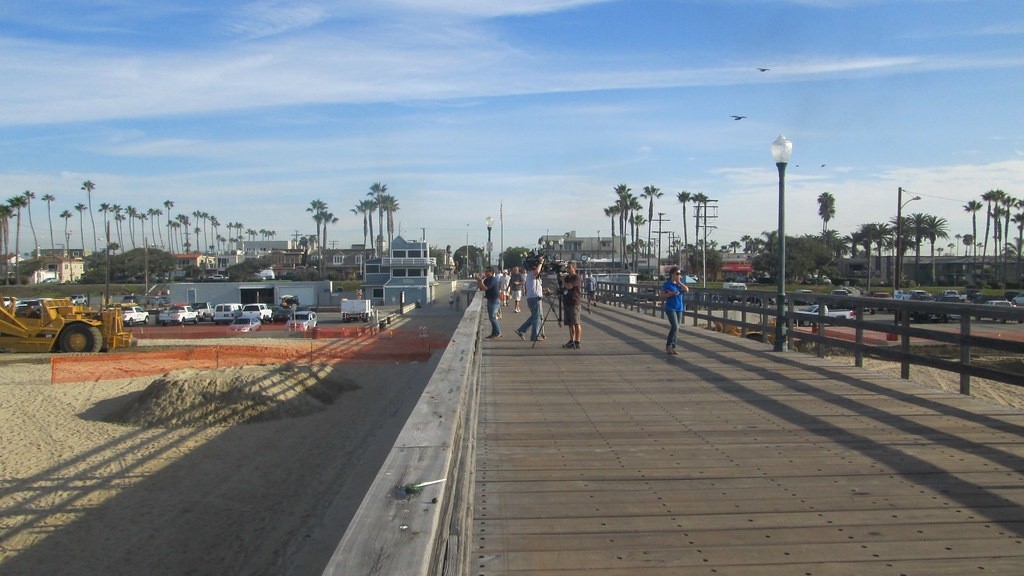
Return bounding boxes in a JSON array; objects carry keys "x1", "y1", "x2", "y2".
[
  {"x1": 487, "y1": 333, "x2": 500, "y2": 339},
  {"x1": 503, "y1": 303, "x2": 509, "y2": 306},
  {"x1": 574, "y1": 340, "x2": 580, "y2": 349},
  {"x1": 667, "y1": 345, "x2": 678, "y2": 355},
  {"x1": 515, "y1": 307, "x2": 521, "y2": 312},
  {"x1": 530, "y1": 335, "x2": 543, "y2": 341},
  {"x1": 515, "y1": 330, "x2": 526, "y2": 341},
  {"x1": 594, "y1": 302, "x2": 597, "y2": 307},
  {"x1": 562, "y1": 340, "x2": 575, "y2": 349}
]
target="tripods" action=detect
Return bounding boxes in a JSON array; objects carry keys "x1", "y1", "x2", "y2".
[{"x1": 532, "y1": 272, "x2": 566, "y2": 349}]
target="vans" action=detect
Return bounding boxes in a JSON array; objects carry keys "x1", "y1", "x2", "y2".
[
  {"x1": 809, "y1": 274, "x2": 832, "y2": 285},
  {"x1": 795, "y1": 289, "x2": 813, "y2": 304},
  {"x1": 240, "y1": 303, "x2": 272, "y2": 324},
  {"x1": 723, "y1": 282, "x2": 747, "y2": 301},
  {"x1": 213, "y1": 302, "x2": 243, "y2": 325}
]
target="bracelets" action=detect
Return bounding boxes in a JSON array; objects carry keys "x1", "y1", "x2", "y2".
[{"x1": 539, "y1": 262, "x2": 543, "y2": 264}]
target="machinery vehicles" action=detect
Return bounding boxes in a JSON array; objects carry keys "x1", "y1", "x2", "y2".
[{"x1": 0, "y1": 295, "x2": 137, "y2": 354}]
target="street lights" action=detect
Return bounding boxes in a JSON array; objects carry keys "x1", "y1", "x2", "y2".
[
  {"x1": 893, "y1": 186, "x2": 922, "y2": 289},
  {"x1": 558, "y1": 236, "x2": 565, "y2": 265},
  {"x1": 56, "y1": 243, "x2": 66, "y2": 259},
  {"x1": 485, "y1": 216, "x2": 494, "y2": 273},
  {"x1": 767, "y1": 132, "x2": 793, "y2": 354}
]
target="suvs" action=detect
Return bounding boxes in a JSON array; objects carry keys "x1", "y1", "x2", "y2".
[
  {"x1": 120, "y1": 306, "x2": 149, "y2": 327},
  {"x1": 285, "y1": 311, "x2": 318, "y2": 333}
]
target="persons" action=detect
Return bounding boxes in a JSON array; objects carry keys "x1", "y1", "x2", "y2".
[
  {"x1": 475, "y1": 266, "x2": 528, "y2": 339},
  {"x1": 557, "y1": 271, "x2": 563, "y2": 285},
  {"x1": 515, "y1": 256, "x2": 544, "y2": 342},
  {"x1": 660, "y1": 267, "x2": 689, "y2": 355},
  {"x1": 559, "y1": 261, "x2": 582, "y2": 349},
  {"x1": 449, "y1": 290, "x2": 460, "y2": 311},
  {"x1": 355, "y1": 288, "x2": 362, "y2": 300},
  {"x1": 585, "y1": 272, "x2": 597, "y2": 314}
]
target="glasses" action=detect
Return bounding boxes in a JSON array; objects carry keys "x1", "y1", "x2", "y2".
[{"x1": 673, "y1": 273, "x2": 682, "y2": 276}]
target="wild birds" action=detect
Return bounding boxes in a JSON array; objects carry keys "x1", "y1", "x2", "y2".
[
  {"x1": 758, "y1": 68, "x2": 770, "y2": 72},
  {"x1": 731, "y1": 116, "x2": 747, "y2": 120}
]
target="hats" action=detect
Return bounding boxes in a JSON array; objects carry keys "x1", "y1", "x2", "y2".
[{"x1": 502, "y1": 269, "x2": 508, "y2": 273}]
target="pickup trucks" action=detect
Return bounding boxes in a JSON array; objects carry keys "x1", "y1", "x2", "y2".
[
  {"x1": 158, "y1": 305, "x2": 200, "y2": 326},
  {"x1": 795, "y1": 303, "x2": 855, "y2": 329},
  {"x1": 191, "y1": 301, "x2": 214, "y2": 322}
]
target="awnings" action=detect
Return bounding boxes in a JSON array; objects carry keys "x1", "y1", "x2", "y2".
[{"x1": 719, "y1": 266, "x2": 753, "y2": 272}]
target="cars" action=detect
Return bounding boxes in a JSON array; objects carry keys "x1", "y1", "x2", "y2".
[
  {"x1": 901, "y1": 290, "x2": 1024, "y2": 324},
  {"x1": 871, "y1": 292, "x2": 893, "y2": 310},
  {"x1": 121, "y1": 295, "x2": 139, "y2": 306},
  {"x1": 829, "y1": 290, "x2": 854, "y2": 310},
  {"x1": 340, "y1": 298, "x2": 374, "y2": 323},
  {"x1": 269, "y1": 304, "x2": 293, "y2": 322},
  {"x1": 2, "y1": 292, "x2": 88, "y2": 319}
]
